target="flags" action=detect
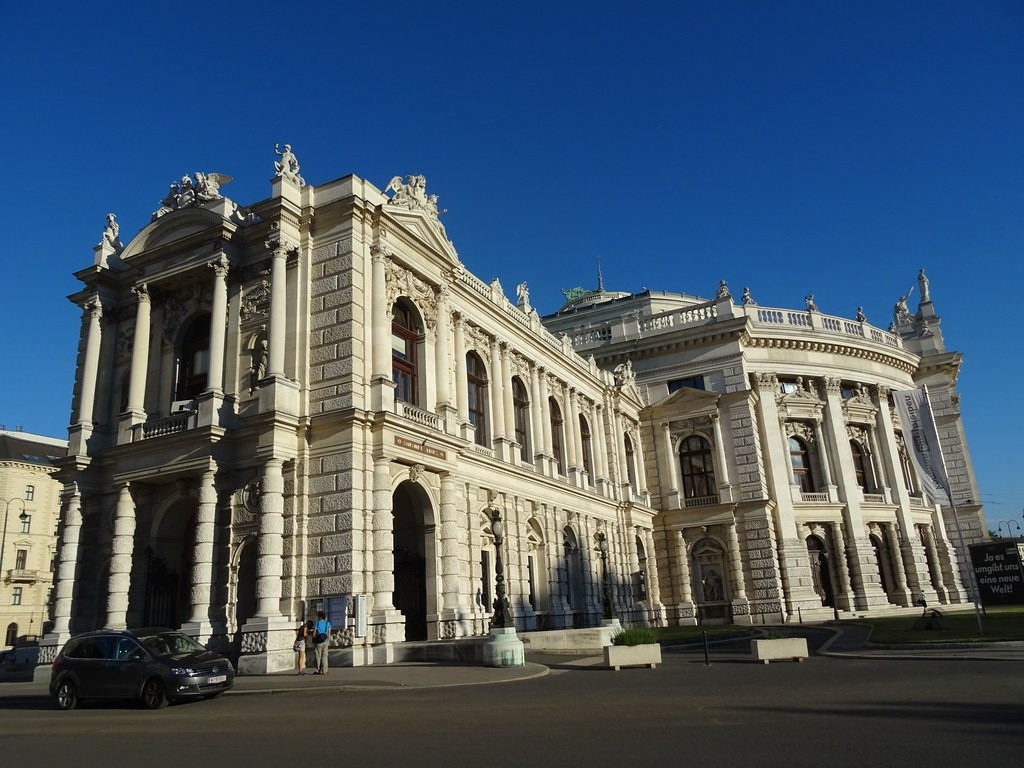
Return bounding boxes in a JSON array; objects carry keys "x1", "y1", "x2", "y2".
[{"x1": 891, "y1": 389, "x2": 952, "y2": 506}]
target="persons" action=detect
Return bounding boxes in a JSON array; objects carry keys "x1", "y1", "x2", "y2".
[
  {"x1": 796, "y1": 377, "x2": 805, "y2": 390},
  {"x1": 921, "y1": 320, "x2": 932, "y2": 335},
  {"x1": 101, "y1": 213, "x2": 118, "y2": 248},
  {"x1": 888, "y1": 322, "x2": 900, "y2": 337},
  {"x1": 894, "y1": 296, "x2": 908, "y2": 324},
  {"x1": 856, "y1": 307, "x2": 867, "y2": 322},
  {"x1": 162, "y1": 172, "x2": 207, "y2": 207},
  {"x1": 853, "y1": 383, "x2": 862, "y2": 395},
  {"x1": 805, "y1": 294, "x2": 819, "y2": 312},
  {"x1": 294, "y1": 620, "x2": 313, "y2": 676},
  {"x1": 274, "y1": 144, "x2": 299, "y2": 177},
  {"x1": 394, "y1": 175, "x2": 447, "y2": 241},
  {"x1": 720, "y1": 280, "x2": 729, "y2": 296},
  {"x1": 517, "y1": 280, "x2": 528, "y2": 302},
  {"x1": 312, "y1": 611, "x2": 331, "y2": 675},
  {"x1": 919, "y1": 590, "x2": 927, "y2": 611},
  {"x1": 742, "y1": 287, "x2": 754, "y2": 305},
  {"x1": 918, "y1": 269, "x2": 929, "y2": 301},
  {"x1": 705, "y1": 570, "x2": 721, "y2": 601}
]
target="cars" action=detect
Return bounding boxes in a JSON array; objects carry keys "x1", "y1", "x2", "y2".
[{"x1": 50, "y1": 625, "x2": 236, "y2": 709}]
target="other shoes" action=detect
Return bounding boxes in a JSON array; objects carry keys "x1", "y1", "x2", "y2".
[
  {"x1": 313, "y1": 671, "x2": 329, "y2": 675},
  {"x1": 297, "y1": 671, "x2": 308, "y2": 676}
]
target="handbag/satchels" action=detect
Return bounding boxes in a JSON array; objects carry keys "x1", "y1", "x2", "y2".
[
  {"x1": 293, "y1": 641, "x2": 301, "y2": 652},
  {"x1": 312, "y1": 633, "x2": 327, "y2": 644}
]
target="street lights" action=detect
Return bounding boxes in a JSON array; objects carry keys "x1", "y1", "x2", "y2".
[
  {"x1": 598, "y1": 532, "x2": 613, "y2": 618},
  {"x1": 0, "y1": 498, "x2": 29, "y2": 579},
  {"x1": 823, "y1": 551, "x2": 839, "y2": 620},
  {"x1": 491, "y1": 509, "x2": 514, "y2": 627},
  {"x1": 999, "y1": 520, "x2": 1021, "y2": 538}
]
